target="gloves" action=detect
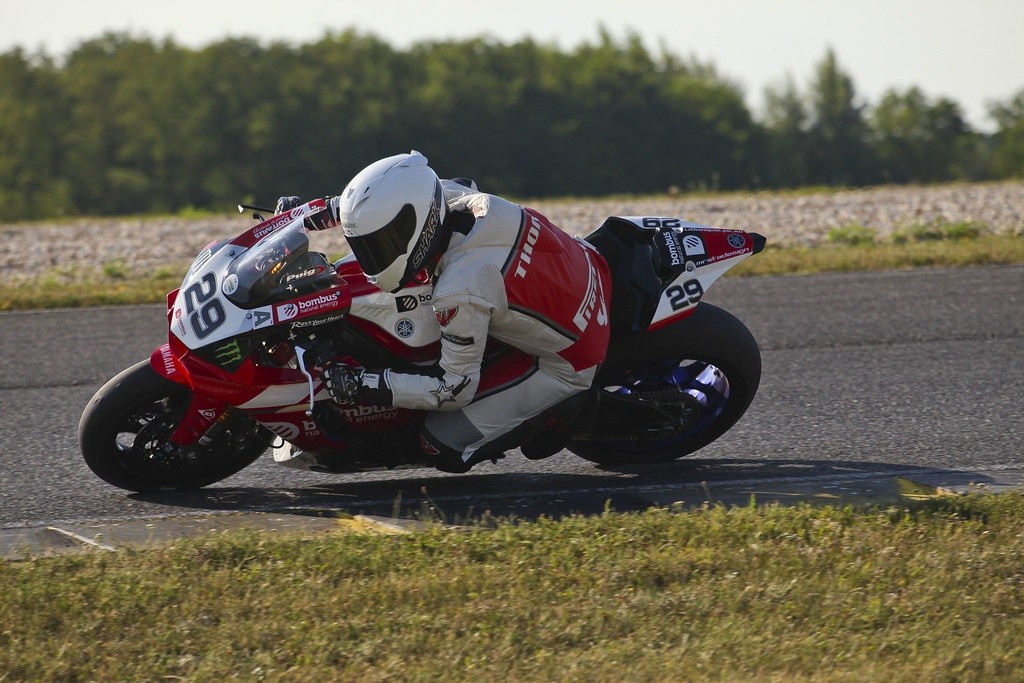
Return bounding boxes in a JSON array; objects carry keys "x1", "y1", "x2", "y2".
[
  {"x1": 273, "y1": 195, "x2": 338, "y2": 231},
  {"x1": 319, "y1": 359, "x2": 396, "y2": 408}
]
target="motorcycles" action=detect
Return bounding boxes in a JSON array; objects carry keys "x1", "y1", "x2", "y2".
[{"x1": 77, "y1": 195, "x2": 768, "y2": 495}]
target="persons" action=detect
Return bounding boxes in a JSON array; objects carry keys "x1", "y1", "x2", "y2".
[{"x1": 274, "y1": 150, "x2": 611, "y2": 474}]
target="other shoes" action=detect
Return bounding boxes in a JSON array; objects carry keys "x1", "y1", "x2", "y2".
[{"x1": 520, "y1": 383, "x2": 601, "y2": 461}]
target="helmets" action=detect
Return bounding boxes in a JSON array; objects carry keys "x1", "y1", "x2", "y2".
[{"x1": 339, "y1": 149, "x2": 451, "y2": 294}]
